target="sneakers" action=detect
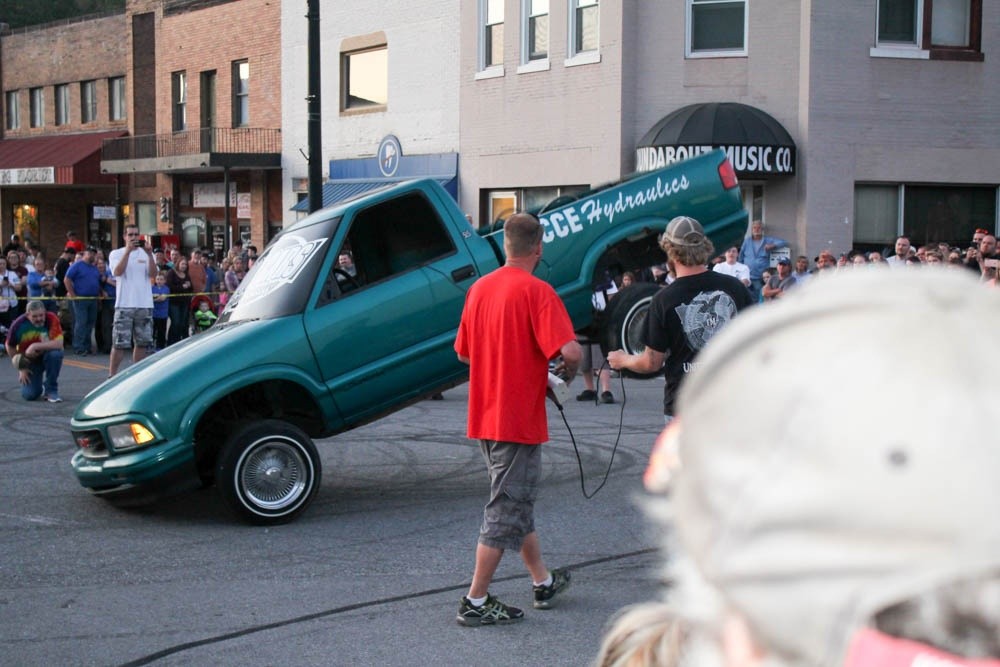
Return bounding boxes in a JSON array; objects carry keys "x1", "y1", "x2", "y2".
[
  {"x1": 533, "y1": 569, "x2": 571, "y2": 609},
  {"x1": 44, "y1": 391, "x2": 62, "y2": 403},
  {"x1": 456, "y1": 591, "x2": 525, "y2": 626}
]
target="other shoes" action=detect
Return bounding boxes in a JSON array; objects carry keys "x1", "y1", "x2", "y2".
[
  {"x1": 74, "y1": 349, "x2": 89, "y2": 356},
  {"x1": 601, "y1": 390, "x2": 614, "y2": 402},
  {"x1": 576, "y1": 390, "x2": 597, "y2": 401}
]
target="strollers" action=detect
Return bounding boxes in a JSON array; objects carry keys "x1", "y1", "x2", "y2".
[{"x1": 188, "y1": 295, "x2": 216, "y2": 336}]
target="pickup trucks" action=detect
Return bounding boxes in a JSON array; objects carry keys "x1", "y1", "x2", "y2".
[{"x1": 71, "y1": 149, "x2": 747, "y2": 528}]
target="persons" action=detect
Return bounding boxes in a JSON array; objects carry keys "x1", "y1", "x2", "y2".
[
  {"x1": 606, "y1": 215, "x2": 754, "y2": 429},
  {"x1": 592, "y1": 263, "x2": 1000, "y2": 667},
  {"x1": 0, "y1": 218, "x2": 1000, "y2": 405},
  {"x1": 453, "y1": 213, "x2": 581, "y2": 628}
]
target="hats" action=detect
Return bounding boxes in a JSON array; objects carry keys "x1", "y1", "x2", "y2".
[
  {"x1": 153, "y1": 248, "x2": 163, "y2": 253},
  {"x1": 642, "y1": 262, "x2": 1000, "y2": 667},
  {"x1": 192, "y1": 248, "x2": 201, "y2": 254},
  {"x1": 64, "y1": 247, "x2": 76, "y2": 254},
  {"x1": 664, "y1": 216, "x2": 704, "y2": 246},
  {"x1": 83, "y1": 245, "x2": 97, "y2": 252},
  {"x1": 777, "y1": 257, "x2": 791, "y2": 264}
]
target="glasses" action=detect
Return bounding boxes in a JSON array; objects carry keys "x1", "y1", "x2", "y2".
[{"x1": 126, "y1": 233, "x2": 140, "y2": 237}]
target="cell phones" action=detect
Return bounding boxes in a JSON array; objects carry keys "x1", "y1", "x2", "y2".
[
  {"x1": 135, "y1": 240, "x2": 145, "y2": 247},
  {"x1": 3, "y1": 277, "x2": 8, "y2": 281}
]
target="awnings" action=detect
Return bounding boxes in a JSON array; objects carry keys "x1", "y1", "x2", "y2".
[
  {"x1": 0, "y1": 131, "x2": 130, "y2": 187},
  {"x1": 290, "y1": 151, "x2": 458, "y2": 215},
  {"x1": 634, "y1": 101, "x2": 798, "y2": 179}
]
